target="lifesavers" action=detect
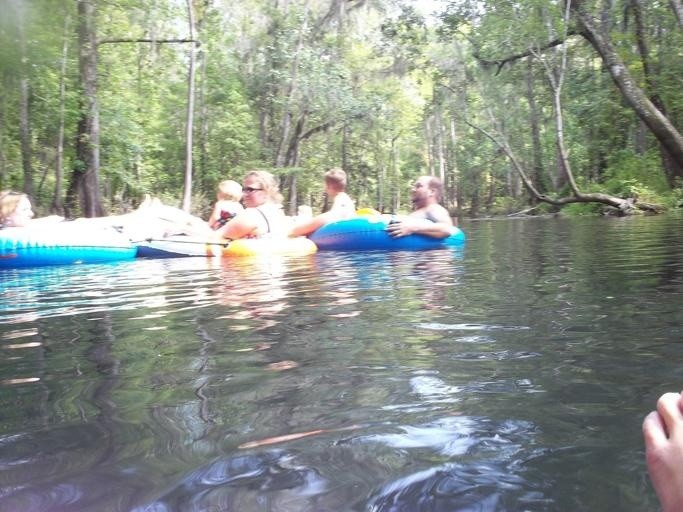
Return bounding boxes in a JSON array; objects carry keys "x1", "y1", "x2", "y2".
[
  {"x1": 287, "y1": 207, "x2": 381, "y2": 233},
  {"x1": 1, "y1": 222, "x2": 139, "y2": 266},
  {"x1": 306, "y1": 212, "x2": 466, "y2": 251},
  {"x1": 224, "y1": 235, "x2": 317, "y2": 259},
  {"x1": 133, "y1": 209, "x2": 221, "y2": 259}
]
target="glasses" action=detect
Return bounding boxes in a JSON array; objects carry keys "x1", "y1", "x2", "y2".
[{"x1": 242, "y1": 188, "x2": 262, "y2": 192}]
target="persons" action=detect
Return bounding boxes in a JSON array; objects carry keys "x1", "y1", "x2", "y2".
[
  {"x1": 641, "y1": 389, "x2": 683, "y2": 512},
  {"x1": 208, "y1": 178, "x2": 246, "y2": 233},
  {"x1": 297, "y1": 166, "x2": 357, "y2": 224},
  {"x1": 298, "y1": 204, "x2": 312, "y2": 218},
  {"x1": 215, "y1": 170, "x2": 287, "y2": 241},
  {"x1": 0, "y1": 189, "x2": 36, "y2": 230},
  {"x1": 386, "y1": 176, "x2": 454, "y2": 238}
]
